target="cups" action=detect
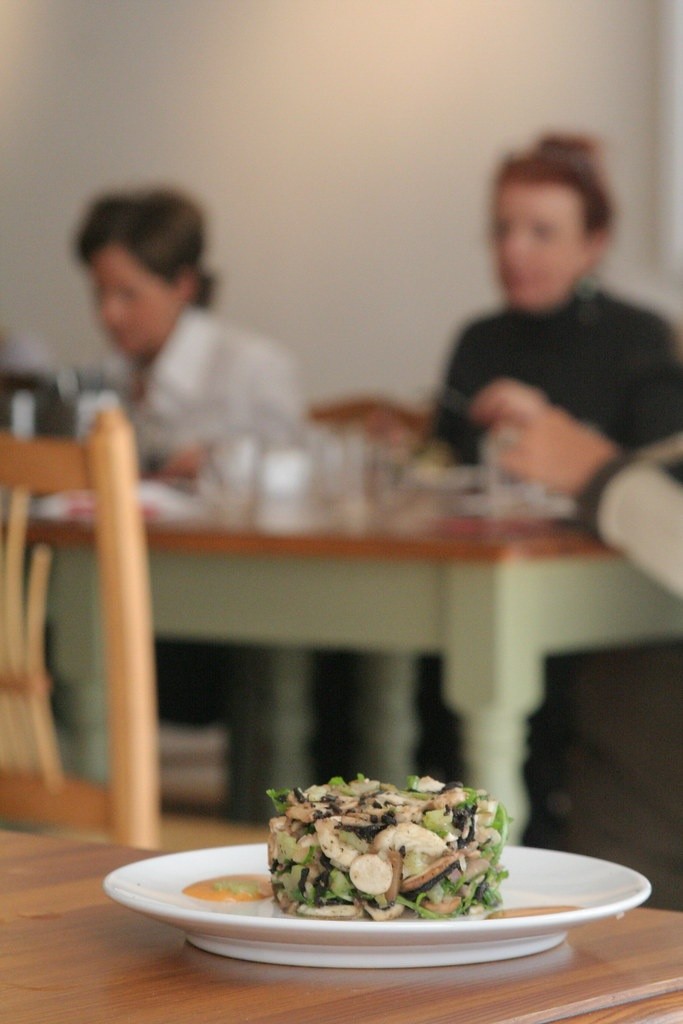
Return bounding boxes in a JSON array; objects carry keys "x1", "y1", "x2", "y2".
[{"x1": 483, "y1": 431, "x2": 545, "y2": 521}]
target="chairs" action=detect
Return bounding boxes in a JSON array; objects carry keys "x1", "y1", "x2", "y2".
[{"x1": 0, "y1": 410, "x2": 273, "y2": 851}]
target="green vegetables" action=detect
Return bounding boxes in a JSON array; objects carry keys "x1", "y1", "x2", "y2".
[{"x1": 265, "y1": 773, "x2": 510, "y2": 919}]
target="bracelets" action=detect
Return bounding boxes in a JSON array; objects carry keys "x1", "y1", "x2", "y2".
[{"x1": 580, "y1": 454, "x2": 632, "y2": 542}]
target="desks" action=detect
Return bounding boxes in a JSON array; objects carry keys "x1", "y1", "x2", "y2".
[
  {"x1": 0, "y1": 512, "x2": 683, "y2": 845},
  {"x1": 0, "y1": 830, "x2": 683, "y2": 1024}
]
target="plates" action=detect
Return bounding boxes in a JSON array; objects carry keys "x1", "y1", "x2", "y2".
[{"x1": 103, "y1": 843, "x2": 652, "y2": 969}]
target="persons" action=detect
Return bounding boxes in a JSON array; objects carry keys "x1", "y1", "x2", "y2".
[
  {"x1": 466, "y1": 381, "x2": 683, "y2": 600},
  {"x1": 417, "y1": 138, "x2": 683, "y2": 846},
  {"x1": 56, "y1": 185, "x2": 297, "y2": 732}
]
